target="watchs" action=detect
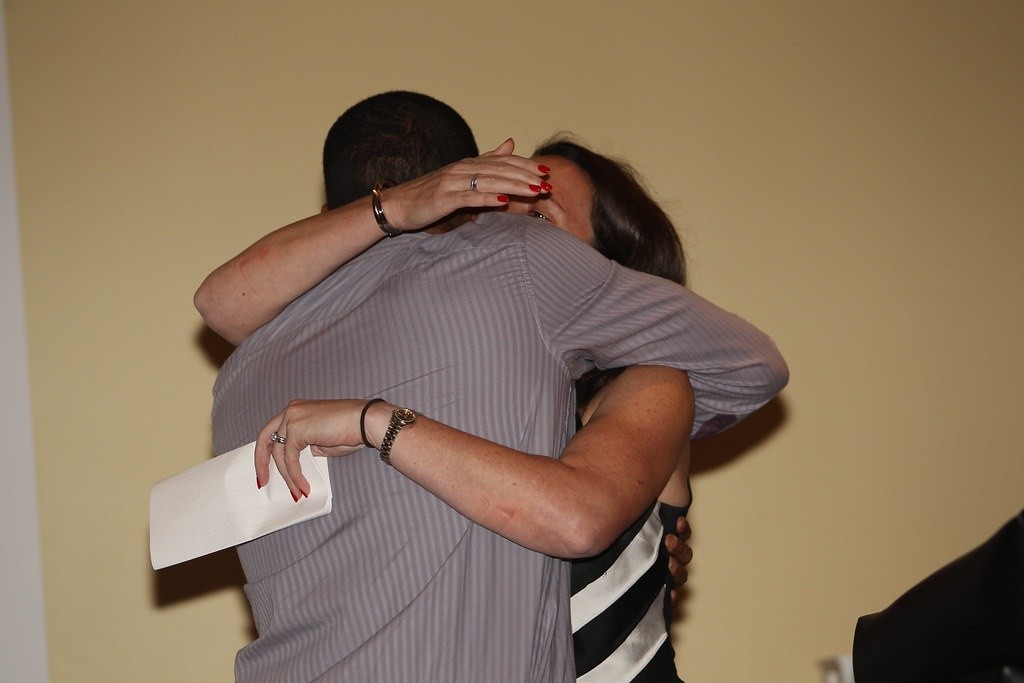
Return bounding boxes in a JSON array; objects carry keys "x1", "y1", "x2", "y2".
[{"x1": 380, "y1": 408, "x2": 423, "y2": 465}]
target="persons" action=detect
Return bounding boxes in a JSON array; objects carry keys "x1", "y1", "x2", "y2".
[
  {"x1": 208, "y1": 91, "x2": 790, "y2": 683},
  {"x1": 192, "y1": 142, "x2": 694, "y2": 683}
]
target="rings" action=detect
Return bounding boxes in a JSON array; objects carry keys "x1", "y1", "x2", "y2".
[
  {"x1": 471, "y1": 173, "x2": 480, "y2": 191},
  {"x1": 271, "y1": 432, "x2": 286, "y2": 443}
]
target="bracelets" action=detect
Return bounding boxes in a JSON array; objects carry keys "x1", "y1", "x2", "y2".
[
  {"x1": 373, "y1": 177, "x2": 401, "y2": 237},
  {"x1": 360, "y1": 398, "x2": 385, "y2": 448}
]
